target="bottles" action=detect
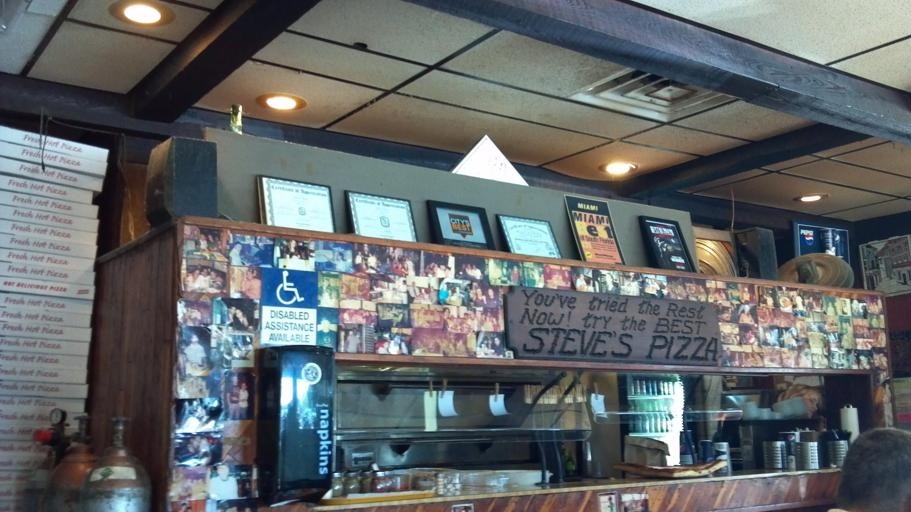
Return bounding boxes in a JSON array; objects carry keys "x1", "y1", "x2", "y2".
[
  {"x1": 333, "y1": 468, "x2": 436, "y2": 497},
  {"x1": 626, "y1": 376, "x2": 677, "y2": 433},
  {"x1": 681, "y1": 429, "x2": 696, "y2": 465}
]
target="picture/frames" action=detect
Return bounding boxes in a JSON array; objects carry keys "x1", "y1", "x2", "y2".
[
  {"x1": 639, "y1": 216, "x2": 696, "y2": 272},
  {"x1": 344, "y1": 189, "x2": 419, "y2": 242},
  {"x1": 426, "y1": 199, "x2": 495, "y2": 249},
  {"x1": 255, "y1": 175, "x2": 335, "y2": 233},
  {"x1": 858, "y1": 234, "x2": 911, "y2": 298},
  {"x1": 495, "y1": 214, "x2": 563, "y2": 258}
]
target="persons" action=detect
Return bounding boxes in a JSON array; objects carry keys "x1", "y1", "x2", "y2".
[{"x1": 170, "y1": 225, "x2": 887, "y2": 512}]
